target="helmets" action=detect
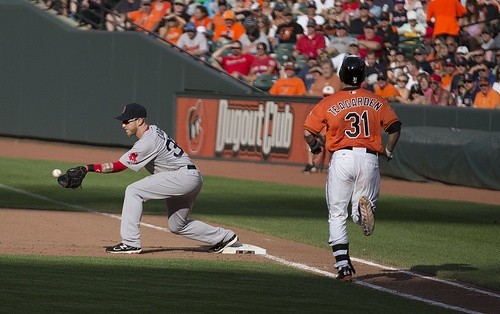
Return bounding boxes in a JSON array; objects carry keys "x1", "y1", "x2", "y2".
[{"x1": 339, "y1": 55, "x2": 365, "y2": 86}]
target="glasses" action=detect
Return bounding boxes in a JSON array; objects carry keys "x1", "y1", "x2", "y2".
[
  {"x1": 397, "y1": 79, "x2": 406, "y2": 83},
  {"x1": 457, "y1": 64, "x2": 466, "y2": 68},
  {"x1": 463, "y1": 80, "x2": 473, "y2": 83},
  {"x1": 122, "y1": 118, "x2": 138, "y2": 124},
  {"x1": 428, "y1": 81, "x2": 437, "y2": 84}
]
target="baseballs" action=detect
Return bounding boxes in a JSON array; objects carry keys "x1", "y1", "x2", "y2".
[{"x1": 52, "y1": 169, "x2": 61, "y2": 177}]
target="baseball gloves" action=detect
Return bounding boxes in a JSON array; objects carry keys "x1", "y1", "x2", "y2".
[{"x1": 58, "y1": 166, "x2": 87, "y2": 189}]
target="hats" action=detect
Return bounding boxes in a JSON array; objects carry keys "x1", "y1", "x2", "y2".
[
  {"x1": 462, "y1": 72, "x2": 474, "y2": 81},
  {"x1": 377, "y1": 71, "x2": 387, "y2": 79},
  {"x1": 185, "y1": 22, "x2": 197, "y2": 31},
  {"x1": 223, "y1": 10, "x2": 234, "y2": 21},
  {"x1": 275, "y1": 2, "x2": 421, "y2": 53},
  {"x1": 141, "y1": 0, "x2": 151, "y2": 5},
  {"x1": 285, "y1": 63, "x2": 295, "y2": 70},
  {"x1": 174, "y1": 0, "x2": 186, "y2": 6},
  {"x1": 456, "y1": 56, "x2": 467, "y2": 65},
  {"x1": 114, "y1": 103, "x2": 146, "y2": 120}
]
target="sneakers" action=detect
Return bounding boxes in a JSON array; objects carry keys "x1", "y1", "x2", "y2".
[
  {"x1": 336, "y1": 268, "x2": 352, "y2": 282},
  {"x1": 213, "y1": 234, "x2": 239, "y2": 253},
  {"x1": 360, "y1": 196, "x2": 375, "y2": 236},
  {"x1": 106, "y1": 243, "x2": 142, "y2": 253}
]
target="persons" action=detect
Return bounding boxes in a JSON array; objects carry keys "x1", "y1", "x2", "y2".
[
  {"x1": 304, "y1": 57, "x2": 402, "y2": 282},
  {"x1": 43, "y1": 0, "x2": 500, "y2": 110},
  {"x1": 58, "y1": 103, "x2": 240, "y2": 253},
  {"x1": 305, "y1": 87, "x2": 335, "y2": 172}
]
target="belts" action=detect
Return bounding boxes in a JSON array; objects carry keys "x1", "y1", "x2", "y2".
[{"x1": 342, "y1": 146, "x2": 377, "y2": 155}]
